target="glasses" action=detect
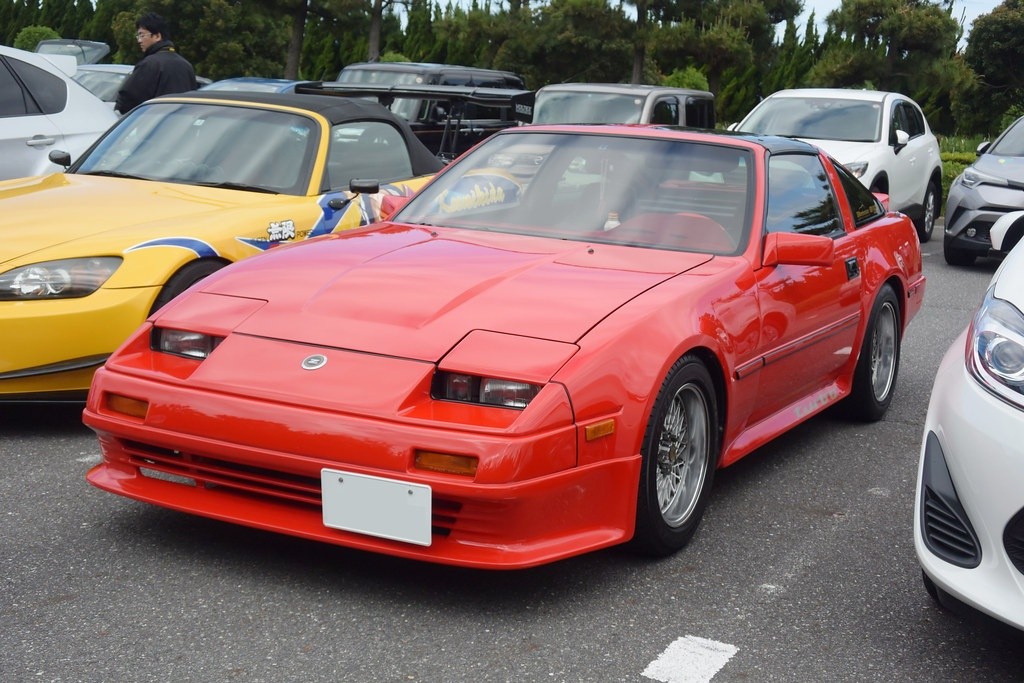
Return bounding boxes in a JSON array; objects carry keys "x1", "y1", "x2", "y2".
[{"x1": 135, "y1": 33, "x2": 153, "y2": 40}]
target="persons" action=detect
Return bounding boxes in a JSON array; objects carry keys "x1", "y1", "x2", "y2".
[{"x1": 114, "y1": 11, "x2": 197, "y2": 116}]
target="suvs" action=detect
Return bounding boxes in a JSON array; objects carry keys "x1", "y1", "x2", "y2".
[
  {"x1": 715, "y1": 86, "x2": 942, "y2": 243},
  {"x1": 479, "y1": 79, "x2": 716, "y2": 218}
]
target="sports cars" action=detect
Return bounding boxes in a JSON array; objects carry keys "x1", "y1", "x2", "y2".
[
  {"x1": 80, "y1": 123, "x2": 928, "y2": 574},
  {"x1": 0, "y1": 88, "x2": 525, "y2": 414}
]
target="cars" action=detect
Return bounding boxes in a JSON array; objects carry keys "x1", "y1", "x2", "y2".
[
  {"x1": 942, "y1": 113, "x2": 1024, "y2": 267},
  {"x1": 0, "y1": 44, "x2": 128, "y2": 185},
  {"x1": 34, "y1": 38, "x2": 535, "y2": 168},
  {"x1": 910, "y1": 235, "x2": 1024, "y2": 639}
]
